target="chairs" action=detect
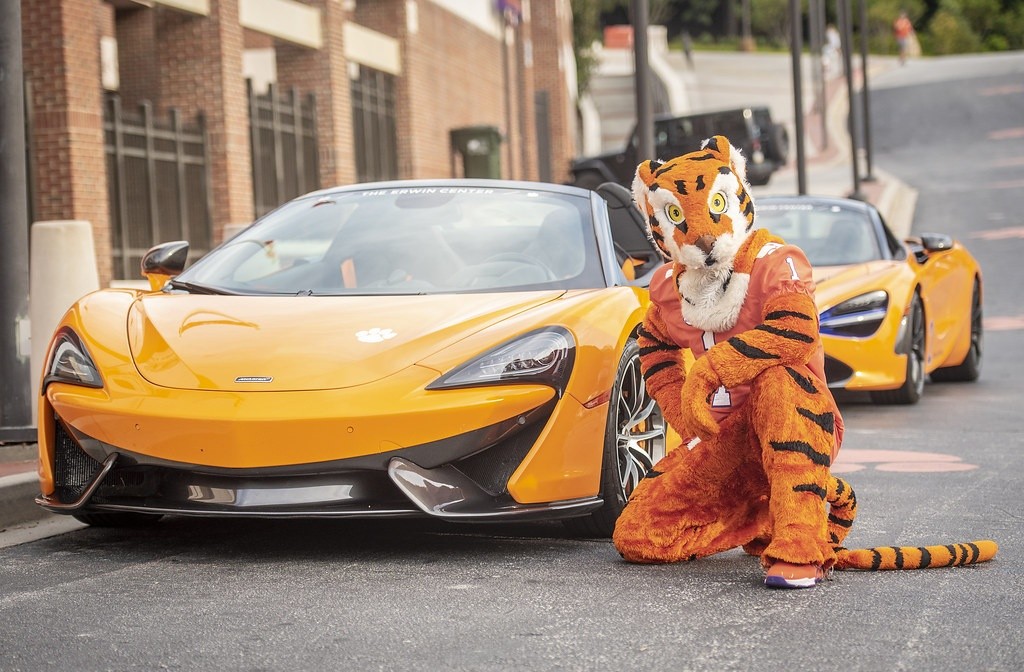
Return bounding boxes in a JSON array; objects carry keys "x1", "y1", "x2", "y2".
[
  {"x1": 526, "y1": 204, "x2": 634, "y2": 280},
  {"x1": 816, "y1": 219, "x2": 873, "y2": 262}
]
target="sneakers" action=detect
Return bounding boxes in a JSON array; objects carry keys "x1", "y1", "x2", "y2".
[{"x1": 764, "y1": 558, "x2": 825, "y2": 587}]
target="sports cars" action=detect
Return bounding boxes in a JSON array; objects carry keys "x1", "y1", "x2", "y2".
[
  {"x1": 32, "y1": 177, "x2": 697, "y2": 542},
  {"x1": 751, "y1": 195, "x2": 986, "y2": 407}
]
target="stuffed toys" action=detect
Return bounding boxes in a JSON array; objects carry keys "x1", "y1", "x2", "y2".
[{"x1": 613, "y1": 134, "x2": 997, "y2": 589}]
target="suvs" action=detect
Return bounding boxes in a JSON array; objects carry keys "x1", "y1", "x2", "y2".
[{"x1": 565, "y1": 105, "x2": 791, "y2": 202}]
